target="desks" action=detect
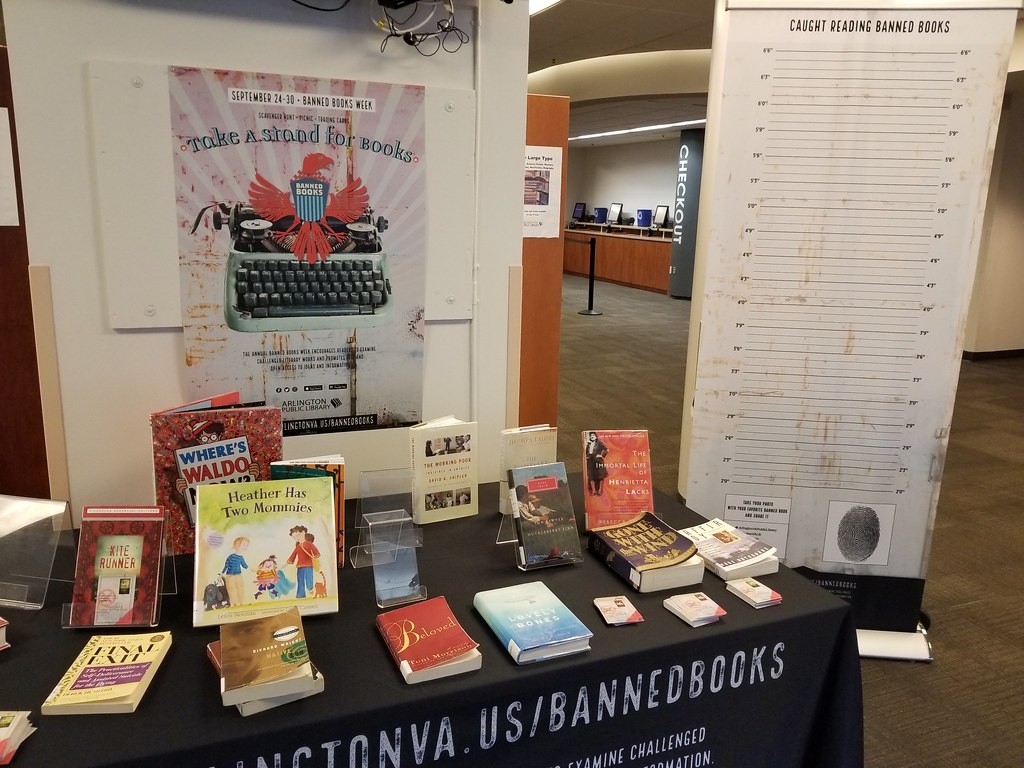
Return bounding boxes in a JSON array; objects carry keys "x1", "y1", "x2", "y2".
[{"x1": 0, "y1": 473, "x2": 866, "y2": 768}]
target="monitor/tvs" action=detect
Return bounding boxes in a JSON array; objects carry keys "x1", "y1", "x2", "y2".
[
  {"x1": 653, "y1": 206, "x2": 669, "y2": 228},
  {"x1": 608, "y1": 203, "x2": 623, "y2": 225},
  {"x1": 572, "y1": 202, "x2": 586, "y2": 222}
]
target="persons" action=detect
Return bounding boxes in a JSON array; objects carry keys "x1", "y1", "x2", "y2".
[
  {"x1": 579, "y1": 423, "x2": 655, "y2": 528},
  {"x1": 516, "y1": 484, "x2": 558, "y2": 528},
  {"x1": 586, "y1": 433, "x2": 609, "y2": 495}
]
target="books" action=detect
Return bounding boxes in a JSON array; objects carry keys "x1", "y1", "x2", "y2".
[
  {"x1": 505, "y1": 463, "x2": 584, "y2": 571},
  {"x1": 410, "y1": 415, "x2": 479, "y2": 523},
  {"x1": 39, "y1": 628, "x2": 174, "y2": 715},
  {"x1": 0, "y1": 612, "x2": 11, "y2": 651},
  {"x1": 376, "y1": 593, "x2": 480, "y2": 684},
  {"x1": 471, "y1": 579, "x2": 594, "y2": 664},
  {"x1": 193, "y1": 452, "x2": 346, "y2": 627},
  {"x1": 0, "y1": 708, "x2": 37, "y2": 763},
  {"x1": 588, "y1": 512, "x2": 706, "y2": 593},
  {"x1": 208, "y1": 606, "x2": 325, "y2": 717},
  {"x1": 677, "y1": 514, "x2": 781, "y2": 578},
  {"x1": 148, "y1": 387, "x2": 285, "y2": 553},
  {"x1": 40, "y1": 627, "x2": 172, "y2": 716},
  {"x1": 67, "y1": 502, "x2": 166, "y2": 627},
  {"x1": 502, "y1": 424, "x2": 583, "y2": 569}
]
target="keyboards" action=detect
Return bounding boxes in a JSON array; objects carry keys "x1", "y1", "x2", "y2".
[{"x1": 236, "y1": 255, "x2": 385, "y2": 315}]
